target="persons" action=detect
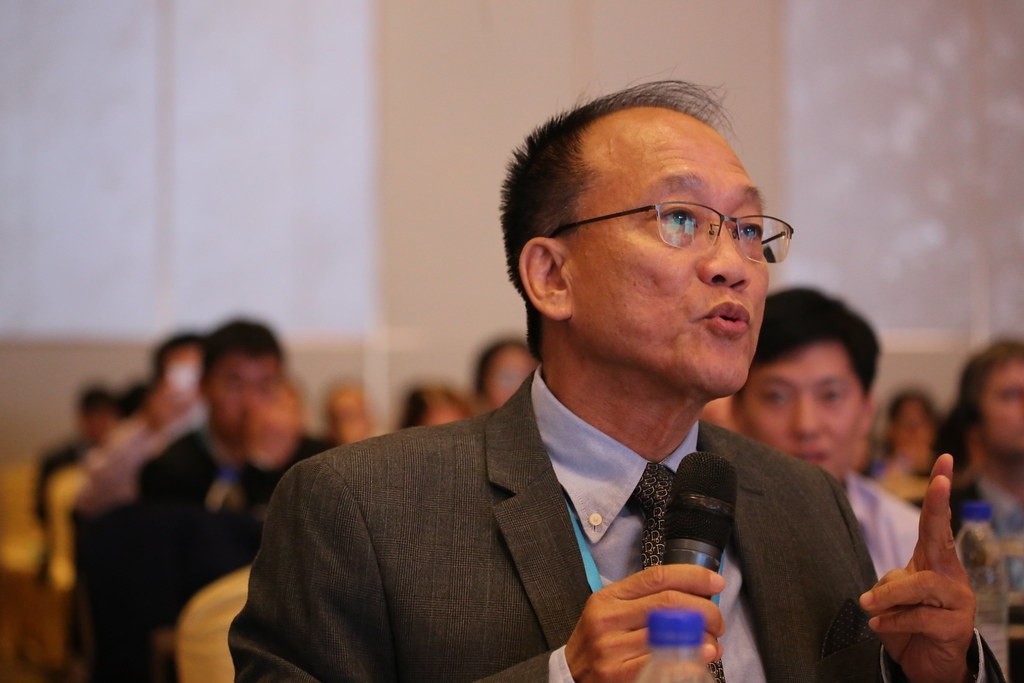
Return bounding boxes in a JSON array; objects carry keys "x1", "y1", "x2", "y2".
[
  {"x1": 225, "y1": 74, "x2": 1010, "y2": 683},
  {"x1": 30, "y1": 317, "x2": 539, "y2": 682},
  {"x1": 704, "y1": 284, "x2": 1024, "y2": 619}
]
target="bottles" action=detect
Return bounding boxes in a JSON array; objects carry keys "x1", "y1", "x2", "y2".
[
  {"x1": 954, "y1": 503, "x2": 1009, "y2": 683},
  {"x1": 636, "y1": 608, "x2": 717, "y2": 683}
]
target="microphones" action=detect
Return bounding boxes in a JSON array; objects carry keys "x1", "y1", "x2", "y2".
[{"x1": 658, "y1": 449, "x2": 741, "y2": 600}]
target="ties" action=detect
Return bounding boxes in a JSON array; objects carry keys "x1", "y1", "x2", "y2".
[{"x1": 626, "y1": 462, "x2": 727, "y2": 683}]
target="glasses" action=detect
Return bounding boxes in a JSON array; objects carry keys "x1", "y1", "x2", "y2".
[{"x1": 548, "y1": 201, "x2": 794, "y2": 265}]
